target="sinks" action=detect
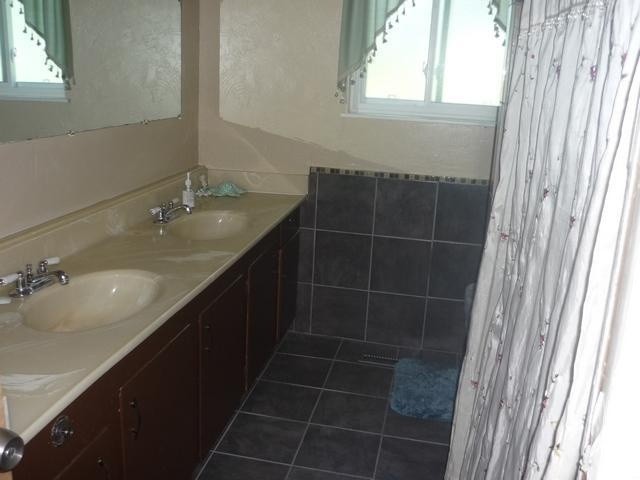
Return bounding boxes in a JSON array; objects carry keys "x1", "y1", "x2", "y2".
[
  {"x1": 165, "y1": 209, "x2": 243, "y2": 243},
  {"x1": 16, "y1": 264, "x2": 163, "y2": 336}
]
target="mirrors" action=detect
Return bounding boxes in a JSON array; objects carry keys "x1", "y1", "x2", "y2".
[{"x1": 1, "y1": 2, "x2": 185, "y2": 146}]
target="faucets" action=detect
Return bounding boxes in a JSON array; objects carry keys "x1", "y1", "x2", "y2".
[
  {"x1": 22, "y1": 262, "x2": 70, "y2": 289},
  {"x1": 149, "y1": 203, "x2": 192, "y2": 222}
]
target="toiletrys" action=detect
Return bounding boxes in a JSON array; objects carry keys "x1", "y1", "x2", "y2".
[{"x1": 182, "y1": 171, "x2": 194, "y2": 208}]
